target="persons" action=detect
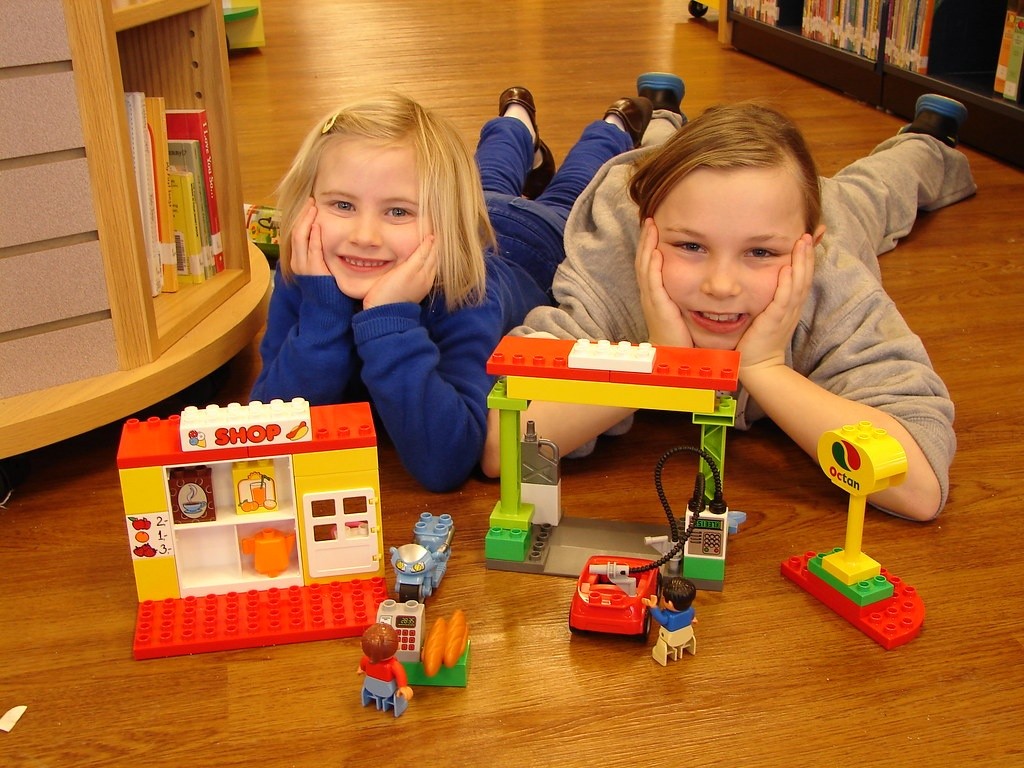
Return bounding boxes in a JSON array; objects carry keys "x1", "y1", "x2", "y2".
[
  {"x1": 642, "y1": 578, "x2": 698, "y2": 665},
  {"x1": 482, "y1": 70, "x2": 978, "y2": 525},
  {"x1": 255, "y1": 86, "x2": 653, "y2": 491},
  {"x1": 357, "y1": 624, "x2": 414, "y2": 717}
]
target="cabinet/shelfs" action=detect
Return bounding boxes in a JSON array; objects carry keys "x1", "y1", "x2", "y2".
[
  {"x1": 720, "y1": 0, "x2": 1024, "y2": 173},
  {"x1": 0, "y1": 2, "x2": 276, "y2": 467}
]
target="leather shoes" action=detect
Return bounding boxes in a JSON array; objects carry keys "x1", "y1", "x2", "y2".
[
  {"x1": 498, "y1": 86, "x2": 555, "y2": 198},
  {"x1": 602, "y1": 97, "x2": 652, "y2": 154}
]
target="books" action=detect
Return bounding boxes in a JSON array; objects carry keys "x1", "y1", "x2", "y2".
[
  {"x1": 734, "y1": 0, "x2": 939, "y2": 74},
  {"x1": 242, "y1": 203, "x2": 281, "y2": 260},
  {"x1": 993, "y1": 4, "x2": 1019, "y2": 94},
  {"x1": 1003, "y1": 14, "x2": 1024, "y2": 102},
  {"x1": 124, "y1": 92, "x2": 226, "y2": 300}
]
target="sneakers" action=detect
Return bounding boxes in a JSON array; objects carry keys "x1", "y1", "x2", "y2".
[
  {"x1": 897, "y1": 95, "x2": 968, "y2": 151},
  {"x1": 636, "y1": 73, "x2": 688, "y2": 128}
]
